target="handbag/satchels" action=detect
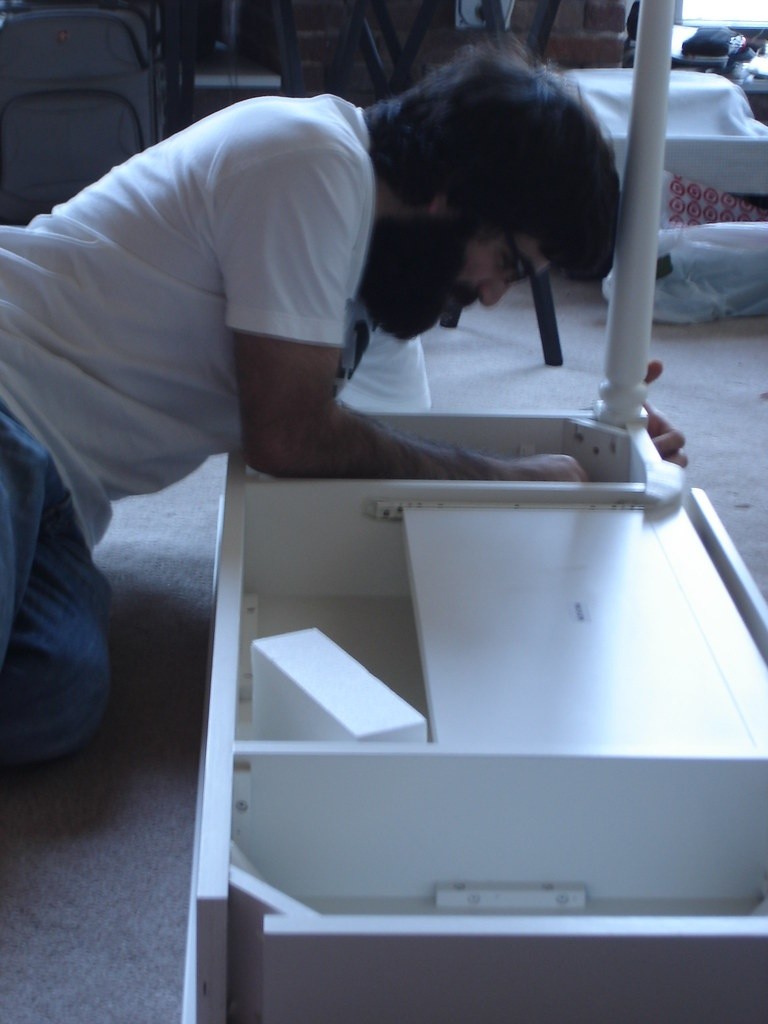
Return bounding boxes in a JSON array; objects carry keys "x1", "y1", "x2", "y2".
[{"x1": 600, "y1": 220, "x2": 768, "y2": 324}]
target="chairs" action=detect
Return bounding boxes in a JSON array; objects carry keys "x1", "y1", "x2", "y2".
[{"x1": 0, "y1": 7, "x2": 164, "y2": 226}]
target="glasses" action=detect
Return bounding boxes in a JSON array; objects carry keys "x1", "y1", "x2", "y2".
[{"x1": 501, "y1": 228, "x2": 534, "y2": 285}]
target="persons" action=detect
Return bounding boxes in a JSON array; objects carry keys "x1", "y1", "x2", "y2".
[{"x1": 0, "y1": 35, "x2": 690, "y2": 774}]
